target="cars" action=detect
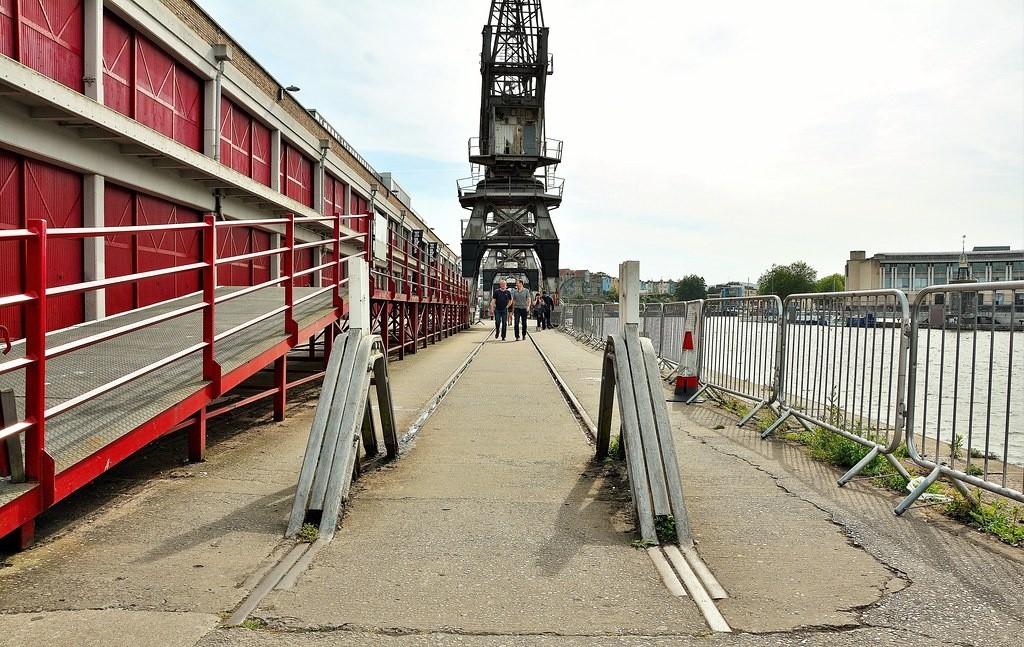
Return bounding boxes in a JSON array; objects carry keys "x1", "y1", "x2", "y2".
[{"x1": 819, "y1": 315, "x2": 833, "y2": 325}]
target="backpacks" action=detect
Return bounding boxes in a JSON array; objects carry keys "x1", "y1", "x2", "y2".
[{"x1": 534, "y1": 310, "x2": 538, "y2": 317}]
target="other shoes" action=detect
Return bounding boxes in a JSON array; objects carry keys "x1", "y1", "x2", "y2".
[
  {"x1": 495, "y1": 330, "x2": 500, "y2": 338},
  {"x1": 515, "y1": 336, "x2": 520, "y2": 341},
  {"x1": 502, "y1": 338, "x2": 504, "y2": 340},
  {"x1": 536, "y1": 327, "x2": 541, "y2": 332},
  {"x1": 523, "y1": 336, "x2": 525, "y2": 340}
]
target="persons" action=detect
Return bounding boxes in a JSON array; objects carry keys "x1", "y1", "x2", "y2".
[
  {"x1": 507, "y1": 305, "x2": 514, "y2": 326},
  {"x1": 492, "y1": 280, "x2": 513, "y2": 342},
  {"x1": 542, "y1": 290, "x2": 554, "y2": 330},
  {"x1": 532, "y1": 292, "x2": 545, "y2": 333},
  {"x1": 512, "y1": 279, "x2": 531, "y2": 342}
]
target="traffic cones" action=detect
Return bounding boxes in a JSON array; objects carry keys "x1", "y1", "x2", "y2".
[{"x1": 666, "y1": 330, "x2": 706, "y2": 403}]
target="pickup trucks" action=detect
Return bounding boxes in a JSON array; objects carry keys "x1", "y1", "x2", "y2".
[{"x1": 826, "y1": 318, "x2": 847, "y2": 327}]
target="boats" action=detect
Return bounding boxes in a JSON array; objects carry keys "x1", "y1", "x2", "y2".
[
  {"x1": 791, "y1": 315, "x2": 821, "y2": 325},
  {"x1": 766, "y1": 316, "x2": 777, "y2": 323},
  {"x1": 741, "y1": 316, "x2": 764, "y2": 322}
]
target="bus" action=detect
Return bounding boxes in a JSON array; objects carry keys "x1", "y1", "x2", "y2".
[{"x1": 791, "y1": 298, "x2": 829, "y2": 310}]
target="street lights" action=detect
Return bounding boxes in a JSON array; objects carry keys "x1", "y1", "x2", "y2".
[{"x1": 769, "y1": 268, "x2": 776, "y2": 295}]
